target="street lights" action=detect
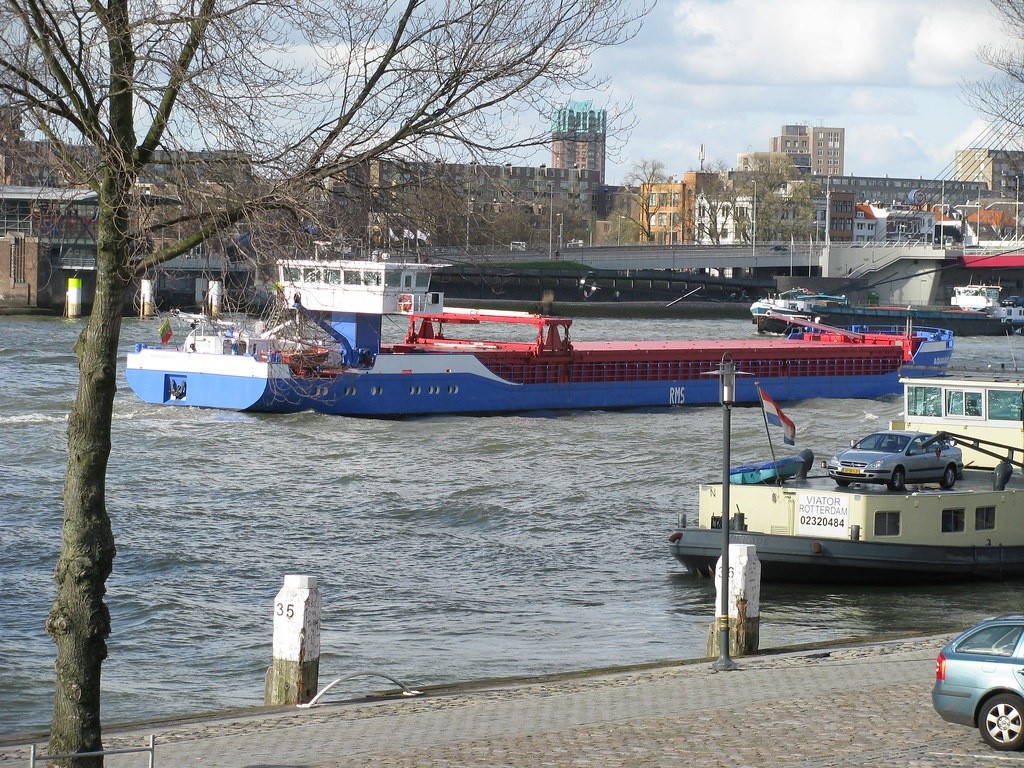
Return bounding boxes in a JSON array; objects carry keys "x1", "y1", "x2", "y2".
[
  {"x1": 975, "y1": 174, "x2": 981, "y2": 244},
  {"x1": 698, "y1": 351, "x2": 755, "y2": 672},
  {"x1": 1014, "y1": 175, "x2": 1020, "y2": 254},
  {"x1": 940, "y1": 178, "x2": 945, "y2": 250},
  {"x1": 550, "y1": 184, "x2": 555, "y2": 259},
  {"x1": 670, "y1": 174, "x2": 677, "y2": 246},
  {"x1": 751, "y1": 180, "x2": 757, "y2": 255},
  {"x1": 825, "y1": 174, "x2": 832, "y2": 241}
]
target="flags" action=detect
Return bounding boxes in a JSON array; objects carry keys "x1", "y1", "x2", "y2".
[
  {"x1": 158, "y1": 319, "x2": 172, "y2": 346},
  {"x1": 386, "y1": 229, "x2": 428, "y2": 244},
  {"x1": 759, "y1": 388, "x2": 796, "y2": 446}
]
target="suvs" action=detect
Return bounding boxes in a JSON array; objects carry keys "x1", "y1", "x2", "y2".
[{"x1": 930, "y1": 615, "x2": 1023, "y2": 752}]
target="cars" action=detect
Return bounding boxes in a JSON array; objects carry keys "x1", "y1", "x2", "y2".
[
  {"x1": 827, "y1": 430, "x2": 964, "y2": 493},
  {"x1": 1002, "y1": 296, "x2": 1023, "y2": 307}
]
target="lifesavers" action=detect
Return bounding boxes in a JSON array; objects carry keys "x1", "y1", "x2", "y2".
[{"x1": 399, "y1": 296, "x2": 412, "y2": 311}]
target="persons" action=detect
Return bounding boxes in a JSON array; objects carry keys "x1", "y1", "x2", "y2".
[{"x1": 254, "y1": 316, "x2": 265, "y2": 335}]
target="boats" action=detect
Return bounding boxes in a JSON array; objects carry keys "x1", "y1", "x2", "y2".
[
  {"x1": 126, "y1": 240, "x2": 954, "y2": 418},
  {"x1": 747, "y1": 275, "x2": 1024, "y2": 336},
  {"x1": 666, "y1": 372, "x2": 1024, "y2": 581}
]
